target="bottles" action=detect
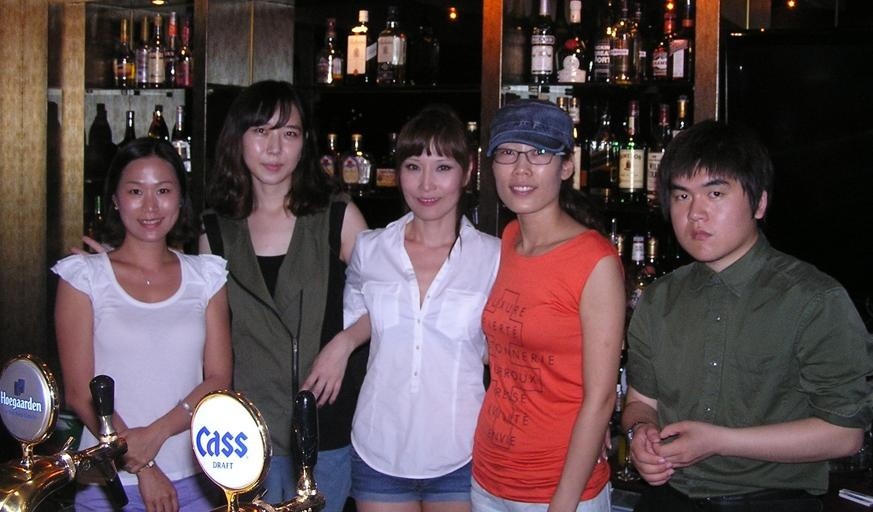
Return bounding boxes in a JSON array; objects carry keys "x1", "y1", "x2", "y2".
[
  {"x1": 86, "y1": 12, "x2": 193, "y2": 245},
  {"x1": 518, "y1": 1, "x2": 700, "y2": 486},
  {"x1": 311, "y1": 5, "x2": 479, "y2": 198}
]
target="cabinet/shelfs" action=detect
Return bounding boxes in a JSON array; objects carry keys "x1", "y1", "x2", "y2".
[{"x1": 59, "y1": 0, "x2": 721, "y2": 492}]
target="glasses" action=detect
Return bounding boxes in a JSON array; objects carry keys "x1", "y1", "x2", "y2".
[{"x1": 493, "y1": 147, "x2": 568, "y2": 166}]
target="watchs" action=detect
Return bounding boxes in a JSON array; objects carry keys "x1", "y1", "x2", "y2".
[{"x1": 626, "y1": 417, "x2": 646, "y2": 444}]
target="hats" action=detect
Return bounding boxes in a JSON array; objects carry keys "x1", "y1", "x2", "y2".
[{"x1": 485, "y1": 98, "x2": 575, "y2": 160}]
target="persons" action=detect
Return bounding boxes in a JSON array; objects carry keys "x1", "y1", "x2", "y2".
[
  {"x1": 620, "y1": 117, "x2": 871, "y2": 511},
  {"x1": 68, "y1": 80, "x2": 372, "y2": 510},
  {"x1": 340, "y1": 106, "x2": 507, "y2": 511},
  {"x1": 470, "y1": 98, "x2": 627, "y2": 511},
  {"x1": 48, "y1": 134, "x2": 229, "y2": 511}
]
